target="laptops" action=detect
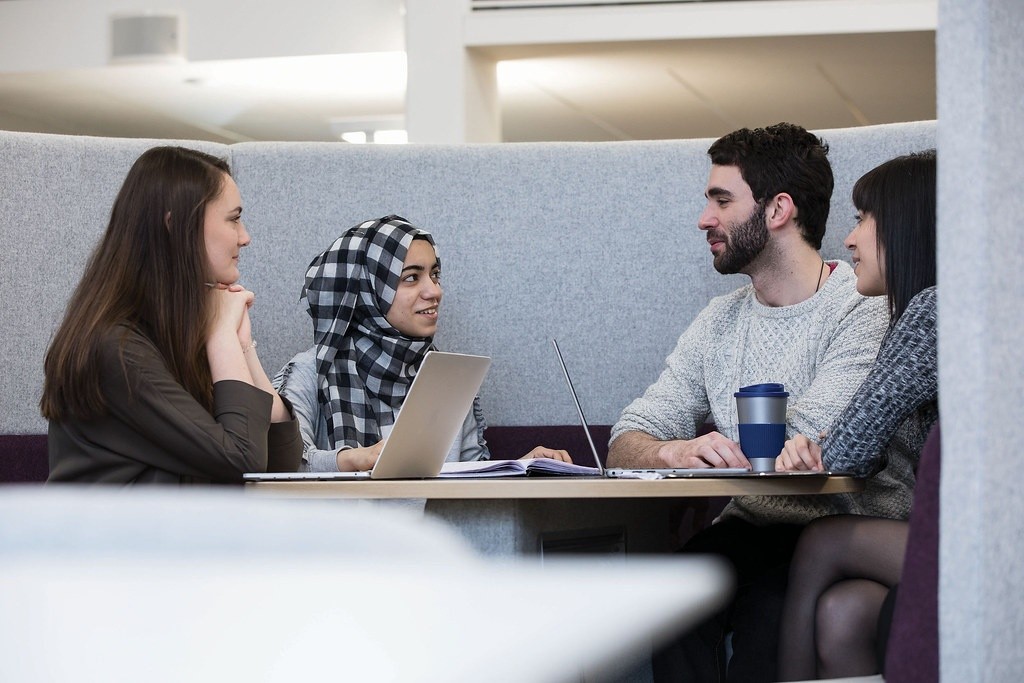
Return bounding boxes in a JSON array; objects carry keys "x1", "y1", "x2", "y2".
[
  {"x1": 553, "y1": 339, "x2": 749, "y2": 479},
  {"x1": 243, "y1": 352, "x2": 492, "y2": 480}
]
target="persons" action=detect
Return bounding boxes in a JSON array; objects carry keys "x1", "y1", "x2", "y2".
[
  {"x1": 273, "y1": 214, "x2": 491, "y2": 479},
  {"x1": 604, "y1": 121, "x2": 937, "y2": 683},
  {"x1": 42, "y1": 144, "x2": 304, "y2": 487}
]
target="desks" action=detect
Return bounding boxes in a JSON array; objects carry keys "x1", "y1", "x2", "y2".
[{"x1": 245, "y1": 477, "x2": 855, "y2": 562}]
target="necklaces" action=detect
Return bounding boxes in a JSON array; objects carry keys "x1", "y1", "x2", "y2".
[{"x1": 816, "y1": 260, "x2": 825, "y2": 292}]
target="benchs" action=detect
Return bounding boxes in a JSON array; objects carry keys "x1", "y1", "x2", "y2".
[{"x1": 0, "y1": 426, "x2": 938, "y2": 683}]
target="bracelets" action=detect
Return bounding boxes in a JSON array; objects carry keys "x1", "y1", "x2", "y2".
[{"x1": 240, "y1": 340, "x2": 257, "y2": 353}]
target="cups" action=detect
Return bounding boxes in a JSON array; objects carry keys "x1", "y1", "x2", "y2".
[{"x1": 734, "y1": 383, "x2": 790, "y2": 472}]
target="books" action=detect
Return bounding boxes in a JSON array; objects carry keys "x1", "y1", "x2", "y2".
[{"x1": 438, "y1": 456, "x2": 607, "y2": 480}]
